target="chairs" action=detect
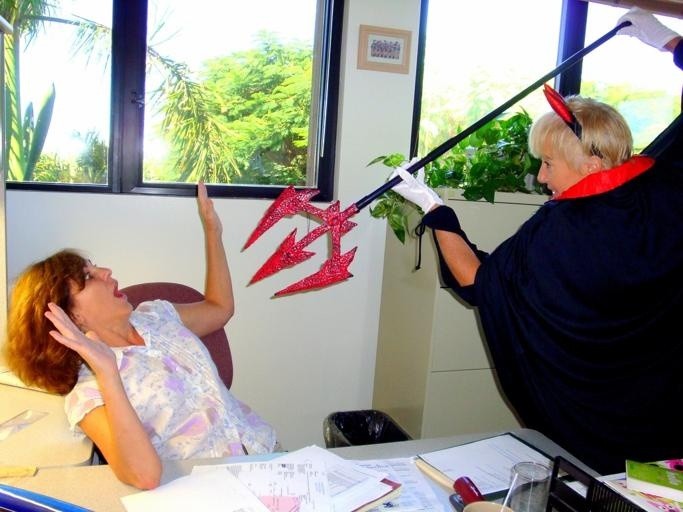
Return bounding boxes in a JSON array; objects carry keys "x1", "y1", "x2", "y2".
[{"x1": 116, "y1": 280, "x2": 234, "y2": 395}]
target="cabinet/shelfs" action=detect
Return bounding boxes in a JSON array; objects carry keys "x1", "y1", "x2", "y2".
[{"x1": 372, "y1": 184, "x2": 547, "y2": 443}]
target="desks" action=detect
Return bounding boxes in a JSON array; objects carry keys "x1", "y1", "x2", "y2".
[{"x1": 0, "y1": 427, "x2": 650, "y2": 512}]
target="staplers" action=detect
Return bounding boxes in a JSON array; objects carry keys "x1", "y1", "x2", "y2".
[{"x1": 450, "y1": 476, "x2": 484, "y2": 512}]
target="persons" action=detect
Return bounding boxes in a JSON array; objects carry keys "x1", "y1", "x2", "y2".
[
  {"x1": 1, "y1": 178, "x2": 284, "y2": 491},
  {"x1": 371, "y1": 40, "x2": 401, "y2": 59},
  {"x1": 389, "y1": 6, "x2": 683, "y2": 476}
]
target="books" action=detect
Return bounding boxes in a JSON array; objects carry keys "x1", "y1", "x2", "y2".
[
  {"x1": 413, "y1": 432, "x2": 569, "y2": 501},
  {"x1": 225, "y1": 445, "x2": 403, "y2": 512},
  {"x1": 626, "y1": 459, "x2": 683, "y2": 502}
]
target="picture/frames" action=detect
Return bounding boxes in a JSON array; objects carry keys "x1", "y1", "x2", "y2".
[{"x1": 353, "y1": 23, "x2": 413, "y2": 77}]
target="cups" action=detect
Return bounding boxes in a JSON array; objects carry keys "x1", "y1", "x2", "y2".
[{"x1": 510, "y1": 460, "x2": 553, "y2": 512}]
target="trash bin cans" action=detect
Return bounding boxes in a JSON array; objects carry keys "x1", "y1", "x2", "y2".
[{"x1": 324, "y1": 409, "x2": 414, "y2": 448}]
